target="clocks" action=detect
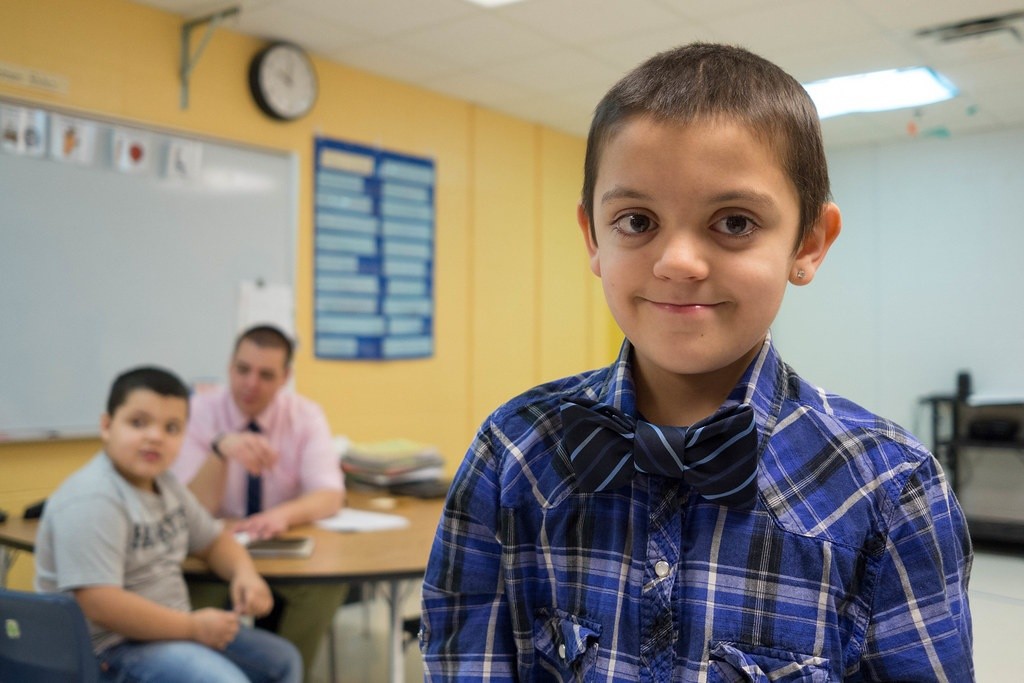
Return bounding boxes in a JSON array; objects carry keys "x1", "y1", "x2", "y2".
[{"x1": 249, "y1": 41, "x2": 320, "y2": 121}]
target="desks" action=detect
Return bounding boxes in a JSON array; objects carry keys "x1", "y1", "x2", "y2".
[{"x1": 0, "y1": 491, "x2": 447, "y2": 683}]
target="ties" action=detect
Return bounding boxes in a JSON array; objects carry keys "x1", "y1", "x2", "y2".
[{"x1": 247, "y1": 422, "x2": 260, "y2": 515}]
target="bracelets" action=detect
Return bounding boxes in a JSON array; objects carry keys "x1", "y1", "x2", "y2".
[{"x1": 212, "y1": 435, "x2": 227, "y2": 460}]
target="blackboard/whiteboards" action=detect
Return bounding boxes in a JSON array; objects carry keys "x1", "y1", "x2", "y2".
[{"x1": 1, "y1": 95, "x2": 301, "y2": 446}]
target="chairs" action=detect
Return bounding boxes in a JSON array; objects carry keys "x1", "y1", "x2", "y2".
[{"x1": 0, "y1": 589, "x2": 102, "y2": 683}]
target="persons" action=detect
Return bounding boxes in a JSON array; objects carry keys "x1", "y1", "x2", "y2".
[
  {"x1": 34, "y1": 365, "x2": 304, "y2": 683},
  {"x1": 169, "y1": 325, "x2": 347, "y2": 683},
  {"x1": 418, "y1": 41, "x2": 977, "y2": 683}
]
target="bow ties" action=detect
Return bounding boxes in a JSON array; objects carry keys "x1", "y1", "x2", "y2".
[{"x1": 560, "y1": 396, "x2": 758, "y2": 512}]
